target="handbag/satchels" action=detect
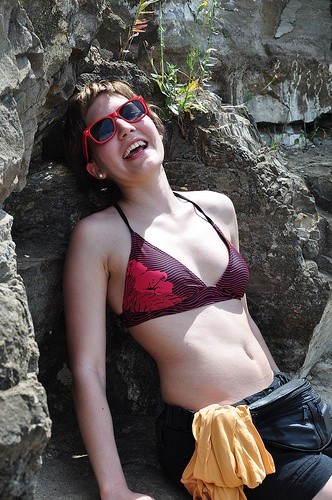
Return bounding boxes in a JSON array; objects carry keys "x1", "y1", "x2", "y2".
[{"x1": 248, "y1": 379, "x2": 332, "y2": 452}]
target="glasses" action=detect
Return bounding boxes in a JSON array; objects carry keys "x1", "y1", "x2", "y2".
[{"x1": 83, "y1": 95, "x2": 151, "y2": 163}]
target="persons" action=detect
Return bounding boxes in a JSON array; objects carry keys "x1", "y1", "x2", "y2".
[{"x1": 63, "y1": 79, "x2": 332, "y2": 500}]
